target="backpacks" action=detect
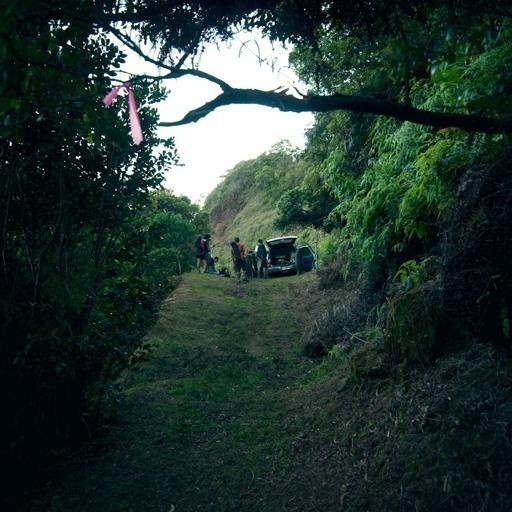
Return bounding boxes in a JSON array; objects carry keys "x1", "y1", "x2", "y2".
[
  {"x1": 256, "y1": 243, "x2": 267, "y2": 260},
  {"x1": 194, "y1": 235, "x2": 206, "y2": 254},
  {"x1": 230, "y1": 241, "x2": 242, "y2": 262}
]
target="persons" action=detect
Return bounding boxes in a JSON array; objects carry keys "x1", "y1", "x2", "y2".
[
  {"x1": 229, "y1": 242, "x2": 242, "y2": 282},
  {"x1": 231, "y1": 237, "x2": 248, "y2": 282},
  {"x1": 194, "y1": 234, "x2": 218, "y2": 274},
  {"x1": 254, "y1": 239, "x2": 270, "y2": 279}
]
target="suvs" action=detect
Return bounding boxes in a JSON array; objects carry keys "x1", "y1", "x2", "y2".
[{"x1": 254, "y1": 236, "x2": 315, "y2": 277}]
